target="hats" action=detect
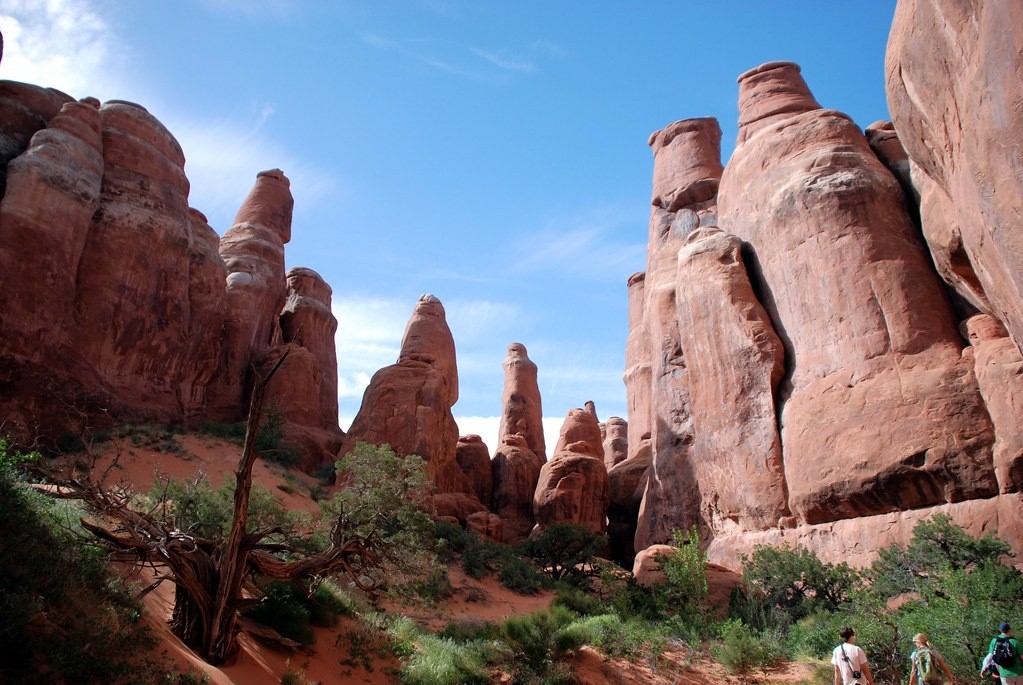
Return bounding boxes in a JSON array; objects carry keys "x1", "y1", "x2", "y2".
[{"x1": 999, "y1": 623, "x2": 1012, "y2": 632}]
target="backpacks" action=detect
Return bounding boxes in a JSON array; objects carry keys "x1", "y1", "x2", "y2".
[
  {"x1": 916, "y1": 651, "x2": 940, "y2": 682},
  {"x1": 992, "y1": 635, "x2": 1017, "y2": 669}
]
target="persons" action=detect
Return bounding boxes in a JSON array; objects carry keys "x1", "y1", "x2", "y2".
[
  {"x1": 832, "y1": 627, "x2": 873, "y2": 685},
  {"x1": 909, "y1": 633, "x2": 954, "y2": 685},
  {"x1": 980, "y1": 623, "x2": 1023, "y2": 685}
]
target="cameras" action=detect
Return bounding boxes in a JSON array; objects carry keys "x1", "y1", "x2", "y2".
[{"x1": 853, "y1": 671, "x2": 861, "y2": 679}]
